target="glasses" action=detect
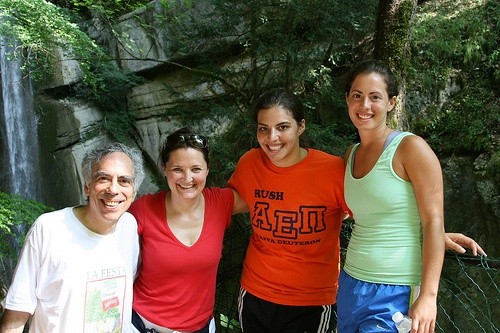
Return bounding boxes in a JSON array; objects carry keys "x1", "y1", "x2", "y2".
[{"x1": 161, "y1": 132, "x2": 209, "y2": 150}]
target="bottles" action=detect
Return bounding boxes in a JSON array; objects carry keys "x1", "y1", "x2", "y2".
[{"x1": 391, "y1": 312, "x2": 414, "y2": 333}]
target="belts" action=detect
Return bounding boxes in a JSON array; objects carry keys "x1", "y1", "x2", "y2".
[{"x1": 136, "y1": 312, "x2": 182, "y2": 333}]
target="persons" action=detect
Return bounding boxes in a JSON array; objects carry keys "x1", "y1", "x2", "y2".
[
  {"x1": 227, "y1": 88, "x2": 487, "y2": 333},
  {"x1": 125, "y1": 127, "x2": 251, "y2": 333},
  {"x1": 337, "y1": 59, "x2": 446, "y2": 333},
  {"x1": 1, "y1": 142, "x2": 141, "y2": 333}
]
